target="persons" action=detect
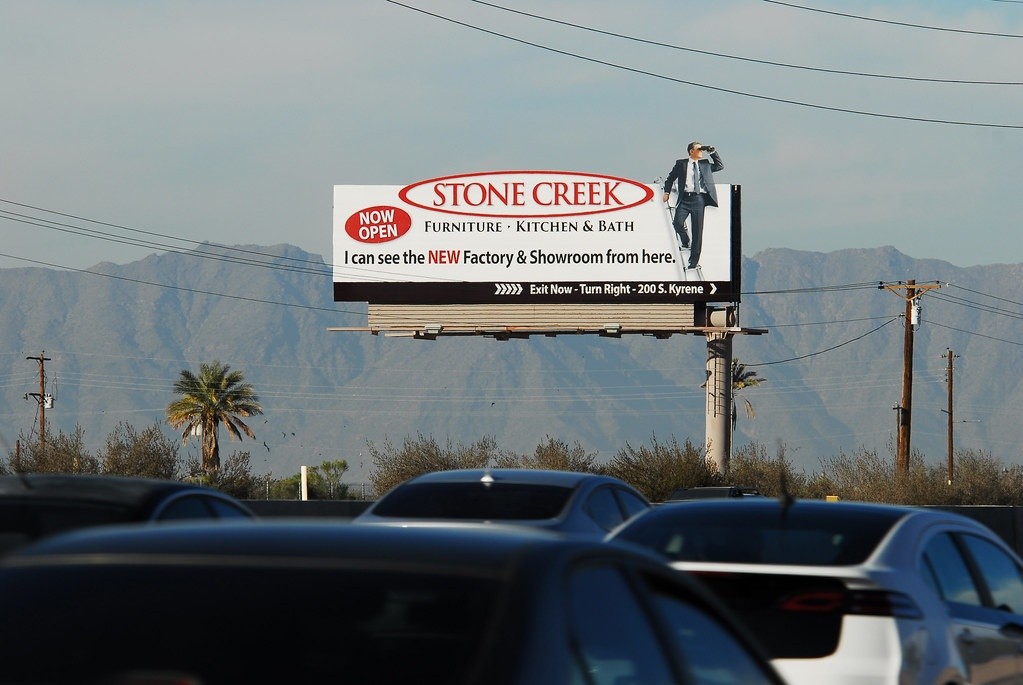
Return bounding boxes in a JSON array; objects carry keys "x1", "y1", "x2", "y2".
[{"x1": 663, "y1": 142, "x2": 725, "y2": 269}]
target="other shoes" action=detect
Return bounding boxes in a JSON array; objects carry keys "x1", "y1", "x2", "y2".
[
  {"x1": 688, "y1": 263, "x2": 697, "y2": 269},
  {"x1": 682, "y1": 240, "x2": 689, "y2": 248}
]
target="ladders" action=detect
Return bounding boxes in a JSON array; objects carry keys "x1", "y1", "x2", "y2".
[{"x1": 653, "y1": 175, "x2": 707, "y2": 282}]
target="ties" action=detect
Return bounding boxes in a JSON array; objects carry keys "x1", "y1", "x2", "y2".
[{"x1": 693, "y1": 162, "x2": 700, "y2": 194}]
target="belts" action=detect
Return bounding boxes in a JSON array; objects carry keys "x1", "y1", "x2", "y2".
[{"x1": 683, "y1": 192, "x2": 704, "y2": 196}]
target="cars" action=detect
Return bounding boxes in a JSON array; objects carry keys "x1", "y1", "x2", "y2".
[
  {"x1": 663, "y1": 485, "x2": 760, "y2": 501},
  {"x1": 351, "y1": 466, "x2": 664, "y2": 537},
  {"x1": 0, "y1": 509, "x2": 789, "y2": 685},
  {"x1": 597, "y1": 495, "x2": 1022, "y2": 685},
  {"x1": 0, "y1": 468, "x2": 263, "y2": 554}
]
